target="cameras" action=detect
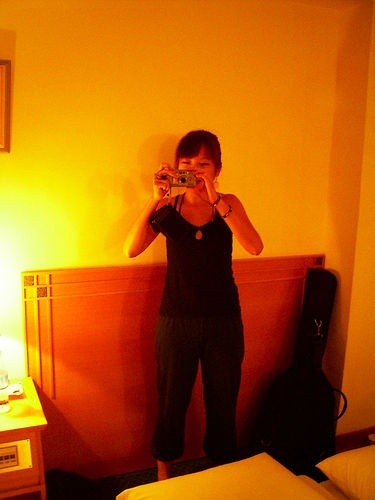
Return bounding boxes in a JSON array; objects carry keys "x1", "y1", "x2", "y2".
[{"x1": 166, "y1": 170, "x2": 196, "y2": 188}]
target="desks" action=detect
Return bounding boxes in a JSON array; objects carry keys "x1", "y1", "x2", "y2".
[{"x1": 1, "y1": 378, "x2": 47, "y2": 499}]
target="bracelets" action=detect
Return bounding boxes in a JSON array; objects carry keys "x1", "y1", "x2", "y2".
[
  {"x1": 210, "y1": 196, "x2": 221, "y2": 208},
  {"x1": 222, "y1": 205, "x2": 233, "y2": 219}
]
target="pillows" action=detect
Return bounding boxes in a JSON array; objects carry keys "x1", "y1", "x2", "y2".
[
  {"x1": 317, "y1": 445, "x2": 375, "y2": 500},
  {"x1": 116, "y1": 453, "x2": 317, "y2": 500}
]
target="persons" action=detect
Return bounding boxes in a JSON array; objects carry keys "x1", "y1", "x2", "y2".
[{"x1": 123, "y1": 129, "x2": 265, "y2": 481}]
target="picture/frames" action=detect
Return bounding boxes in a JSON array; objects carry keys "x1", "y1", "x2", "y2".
[{"x1": 0, "y1": 59, "x2": 11, "y2": 152}]
